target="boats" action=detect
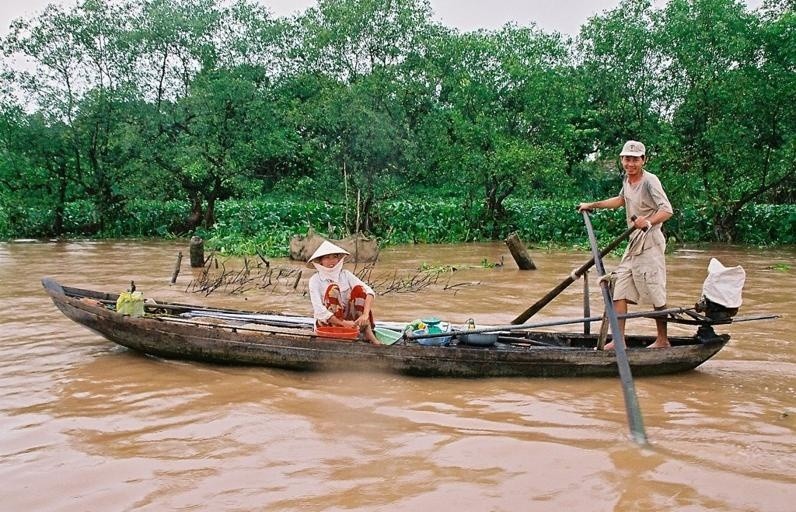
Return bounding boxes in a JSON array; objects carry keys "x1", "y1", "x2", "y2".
[{"x1": 41, "y1": 257, "x2": 745, "y2": 377}]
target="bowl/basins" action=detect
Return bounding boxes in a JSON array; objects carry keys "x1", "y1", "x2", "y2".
[
  {"x1": 453, "y1": 328, "x2": 500, "y2": 346},
  {"x1": 373, "y1": 327, "x2": 402, "y2": 345},
  {"x1": 314, "y1": 326, "x2": 360, "y2": 340},
  {"x1": 412, "y1": 327, "x2": 453, "y2": 345}
]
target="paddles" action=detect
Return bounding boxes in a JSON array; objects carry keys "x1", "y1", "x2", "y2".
[
  {"x1": 511, "y1": 214, "x2": 647, "y2": 324},
  {"x1": 575, "y1": 204, "x2": 649, "y2": 446}
]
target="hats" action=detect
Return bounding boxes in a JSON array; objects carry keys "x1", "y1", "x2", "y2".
[
  {"x1": 305, "y1": 240, "x2": 350, "y2": 269},
  {"x1": 619, "y1": 140, "x2": 645, "y2": 161}
]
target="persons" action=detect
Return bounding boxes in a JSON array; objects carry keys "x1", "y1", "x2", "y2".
[
  {"x1": 306, "y1": 239, "x2": 382, "y2": 345},
  {"x1": 577, "y1": 140, "x2": 674, "y2": 351}
]
